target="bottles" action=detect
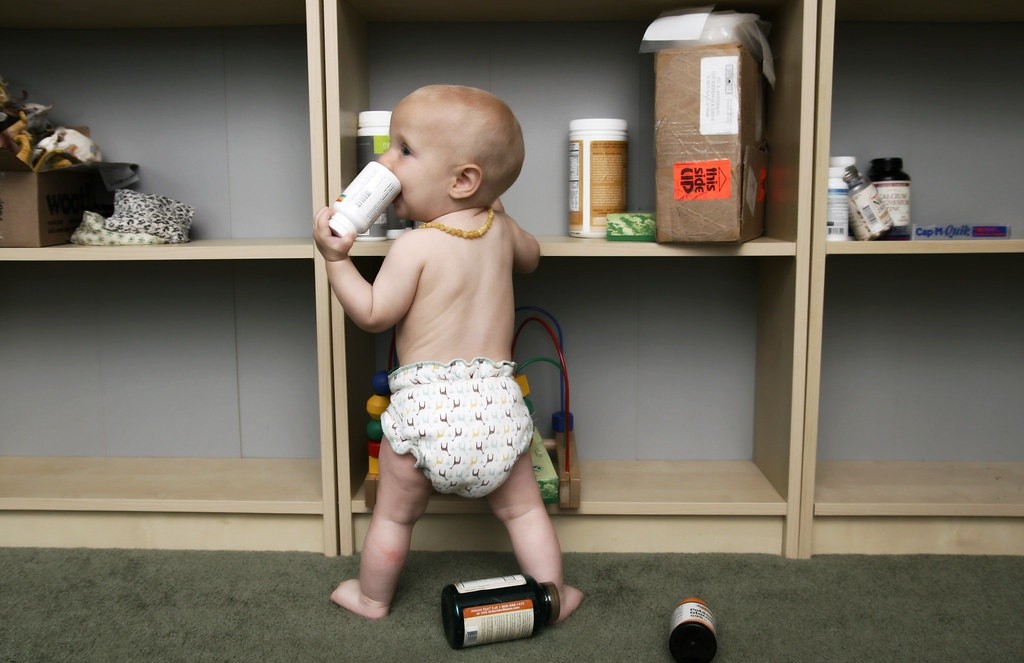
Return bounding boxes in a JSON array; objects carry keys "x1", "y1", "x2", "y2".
[
  {"x1": 441, "y1": 573, "x2": 563, "y2": 650},
  {"x1": 819, "y1": 150, "x2": 916, "y2": 240},
  {"x1": 567, "y1": 116, "x2": 631, "y2": 238},
  {"x1": 324, "y1": 113, "x2": 413, "y2": 247},
  {"x1": 668, "y1": 596, "x2": 719, "y2": 662}
]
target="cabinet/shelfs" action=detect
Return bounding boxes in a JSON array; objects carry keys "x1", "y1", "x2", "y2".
[{"x1": 1, "y1": 1, "x2": 1023, "y2": 551}]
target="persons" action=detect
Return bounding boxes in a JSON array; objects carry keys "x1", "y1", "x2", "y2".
[{"x1": 312, "y1": 84, "x2": 585, "y2": 624}]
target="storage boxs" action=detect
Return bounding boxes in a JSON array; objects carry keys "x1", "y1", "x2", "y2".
[{"x1": 0, "y1": 127, "x2": 95, "y2": 247}]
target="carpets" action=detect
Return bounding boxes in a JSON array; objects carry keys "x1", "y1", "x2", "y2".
[{"x1": 0, "y1": 547, "x2": 1024, "y2": 663}]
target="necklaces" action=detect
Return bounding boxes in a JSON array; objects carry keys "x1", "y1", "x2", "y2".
[{"x1": 414, "y1": 206, "x2": 495, "y2": 240}]
